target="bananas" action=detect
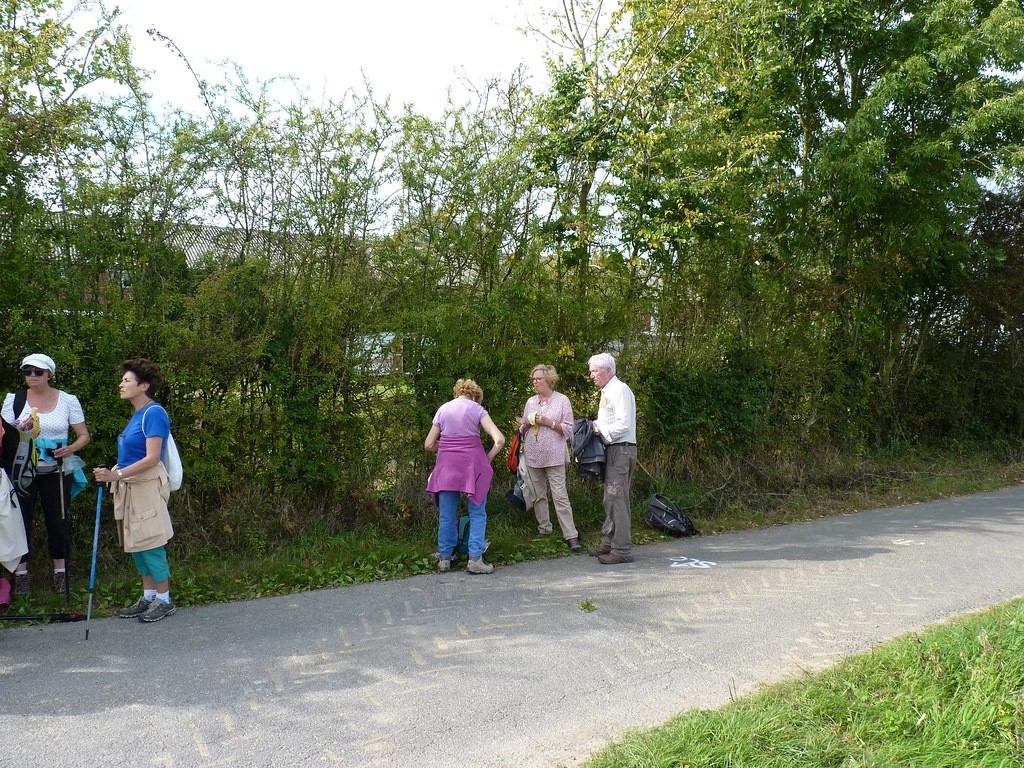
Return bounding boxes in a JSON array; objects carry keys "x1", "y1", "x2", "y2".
[{"x1": 29, "y1": 407, "x2": 41, "y2": 438}]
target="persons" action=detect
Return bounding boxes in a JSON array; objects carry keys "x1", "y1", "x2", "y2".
[
  {"x1": 586, "y1": 352, "x2": 636, "y2": 564},
  {"x1": 423, "y1": 379, "x2": 506, "y2": 573},
  {"x1": 0, "y1": 353, "x2": 90, "y2": 598},
  {"x1": 520, "y1": 365, "x2": 582, "y2": 551},
  {"x1": 91, "y1": 357, "x2": 176, "y2": 622}
]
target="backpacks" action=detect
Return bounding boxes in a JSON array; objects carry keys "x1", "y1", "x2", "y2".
[
  {"x1": 0, "y1": 415, "x2": 38, "y2": 497},
  {"x1": 646, "y1": 493, "x2": 695, "y2": 539},
  {"x1": 142, "y1": 405, "x2": 182, "y2": 492},
  {"x1": 454, "y1": 516, "x2": 471, "y2": 557}
]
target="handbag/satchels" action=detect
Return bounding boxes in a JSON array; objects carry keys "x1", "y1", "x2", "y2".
[{"x1": 506, "y1": 428, "x2": 522, "y2": 476}]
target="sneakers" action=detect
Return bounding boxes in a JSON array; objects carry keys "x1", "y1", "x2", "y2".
[
  {"x1": 139, "y1": 597, "x2": 176, "y2": 622},
  {"x1": 466, "y1": 555, "x2": 494, "y2": 574},
  {"x1": 589, "y1": 546, "x2": 610, "y2": 556},
  {"x1": 118, "y1": 596, "x2": 154, "y2": 618},
  {"x1": 12, "y1": 573, "x2": 31, "y2": 599},
  {"x1": 436, "y1": 558, "x2": 450, "y2": 571},
  {"x1": 597, "y1": 553, "x2": 634, "y2": 564},
  {"x1": 567, "y1": 537, "x2": 581, "y2": 550},
  {"x1": 52, "y1": 573, "x2": 69, "y2": 599}
]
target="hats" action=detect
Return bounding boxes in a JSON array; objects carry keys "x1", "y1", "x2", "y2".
[{"x1": 19, "y1": 353, "x2": 56, "y2": 376}]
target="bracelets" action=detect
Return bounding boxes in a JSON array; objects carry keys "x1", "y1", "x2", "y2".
[{"x1": 117, "y1": 470, "x2": 122, "y2": 479}]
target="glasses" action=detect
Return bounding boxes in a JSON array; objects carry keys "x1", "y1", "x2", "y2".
[
  {"x1": 21, "y1": 366, "x2": 49, "y2": 377},
  {"x1": 532, "y1": 377, "x2": 546, "y2": 382}
]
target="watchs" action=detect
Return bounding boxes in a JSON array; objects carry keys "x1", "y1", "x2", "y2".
[{"x1": 550, "y1": 421, "x2": 556, "y2": 430}]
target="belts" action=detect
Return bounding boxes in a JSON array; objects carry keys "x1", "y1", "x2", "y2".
[{"x1": 607, "y1": 443, "x2": 636, "y2": 447}]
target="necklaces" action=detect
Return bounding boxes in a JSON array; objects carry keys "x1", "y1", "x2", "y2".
[{"x1": 144, "y1": 399, "x2": 153, "y2": 406}]
